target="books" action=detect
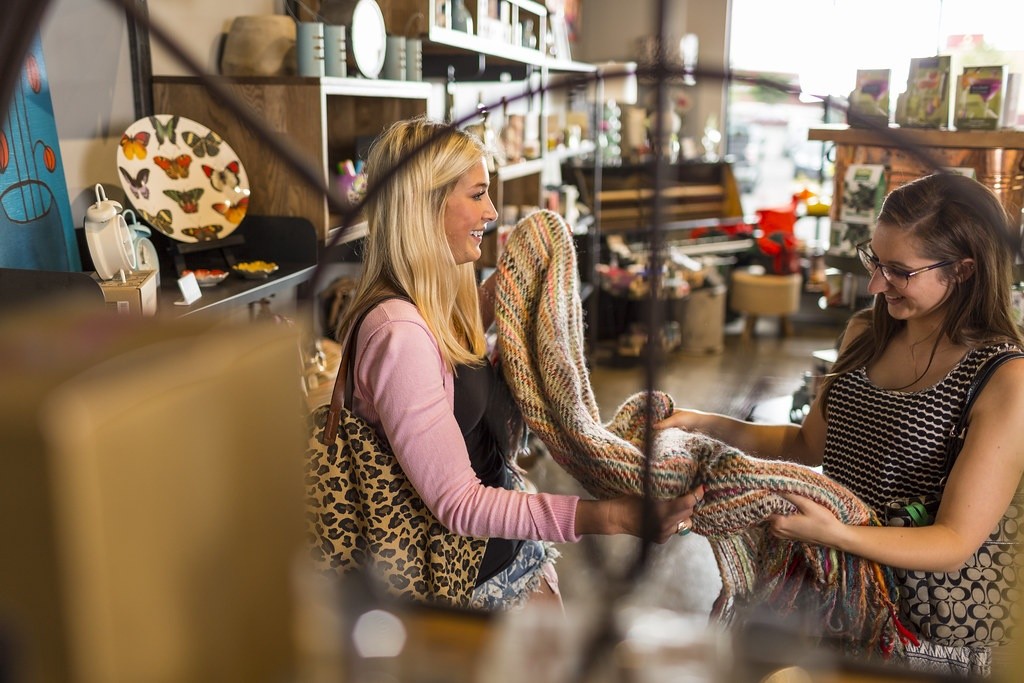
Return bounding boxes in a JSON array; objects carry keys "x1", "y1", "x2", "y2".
[{"x1": 856, "y1": 56, "x2": 1003, "y2": 128}]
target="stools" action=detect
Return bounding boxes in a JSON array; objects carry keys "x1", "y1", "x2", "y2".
[{"x1": 728, "y1": 267, "x2": 800, "y2": 341}]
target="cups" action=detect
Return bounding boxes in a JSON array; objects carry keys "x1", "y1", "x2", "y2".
[
  {"x1": 384, "y1": 35, "x2": 407, "y2": 80},
  {"x1": 325, "y1": 26, "x2": 348, "y2": 77},
  {"x1": 334, "y1": 174, "x2": 368, "y2": 214},
  {"x1": 405, "y1": 40, "x2": 423, "y2": 82},
  {"x1": 296, "y1": 23, "x2": 326, "y2": 78}
]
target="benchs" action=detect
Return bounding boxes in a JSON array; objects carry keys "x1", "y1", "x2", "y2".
[{"x1": 560, "y1": 159, "x2": 752, "y2": 337}]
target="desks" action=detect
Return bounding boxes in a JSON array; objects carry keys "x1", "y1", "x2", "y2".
[{"x1": 809, "y1": 123, "x2": 1024, "y2": 286}]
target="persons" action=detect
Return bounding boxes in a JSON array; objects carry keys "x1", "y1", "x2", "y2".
[
  {"x1": 336, "y1": 120, "x2": 704, "y2": 614},
  {"x1": 654, "y1": 171, "x2": 1023, "y2": 675}
]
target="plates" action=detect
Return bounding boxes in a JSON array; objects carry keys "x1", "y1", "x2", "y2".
[
  {"x1": 232, "y1": 264, "x2": 279, "y2": 279},
  {"x1": 180, "y1": 272, "x2": 230, "y2": 287},
  {"x1": 116, "y1": 113, "x2": 250, "y2": 243},
  {"x1": 352, "y1": 0, "x2": 387, "y2": 79}
]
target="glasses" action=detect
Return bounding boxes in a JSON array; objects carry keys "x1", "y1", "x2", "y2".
[{"x1": 859, "y1": 242, "x2": 957, "y2": 289}]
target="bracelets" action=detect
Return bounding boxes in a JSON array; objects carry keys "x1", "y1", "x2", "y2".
[{"x1": 481, "y1": 285, "x2": 494, "y2": 304}]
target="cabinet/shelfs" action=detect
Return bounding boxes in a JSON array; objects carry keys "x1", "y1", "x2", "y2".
[{"x1": 153, "y1": 0, "x2": 604, "y2": 357}]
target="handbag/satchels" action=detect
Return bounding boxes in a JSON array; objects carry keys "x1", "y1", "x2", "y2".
[
  {"x1": 297, "y1": 294, "x2": 488, "y2": 612},
  {"x1": 887, "y1": 351, "x2": 1022, "y2": 645}
]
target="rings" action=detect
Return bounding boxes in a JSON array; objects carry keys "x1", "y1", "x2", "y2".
[
  {"x1": 691, "y1": 490, "x2": 699, "y2": 504},
  {"x1": 679, "y1": 522, "x2": 690, "y2": 536}
]
